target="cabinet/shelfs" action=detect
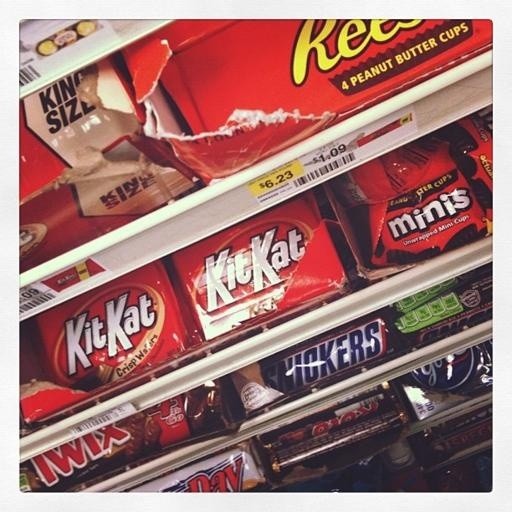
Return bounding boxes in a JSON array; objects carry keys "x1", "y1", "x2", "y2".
[{"x1": 21, "y1": 19, "x2": 492, "y2": 492}]
